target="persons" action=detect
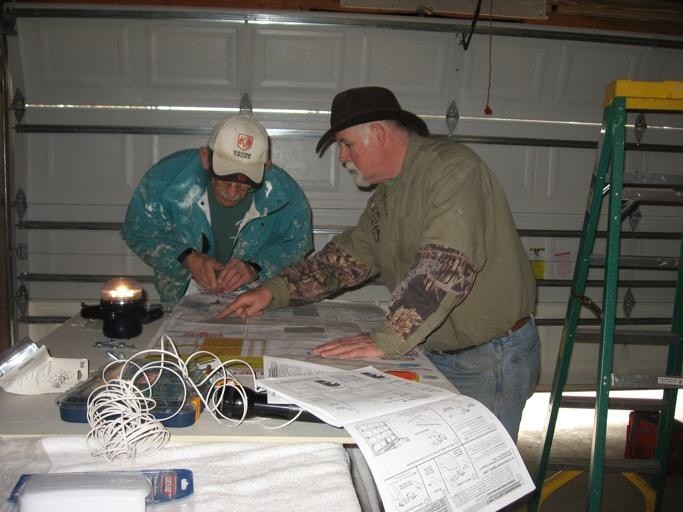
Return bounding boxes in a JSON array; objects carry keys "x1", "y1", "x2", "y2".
[
  {"x1": 120, "y1": 112, "x2": 315, "y2": 304},
  {"x1": 213, "y1": 85, "x2": 542, "y2": 446}
]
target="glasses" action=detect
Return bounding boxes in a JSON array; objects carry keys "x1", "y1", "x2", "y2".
[{"x1": 211, "y1": 174, "x2": 260, "y2": 188}]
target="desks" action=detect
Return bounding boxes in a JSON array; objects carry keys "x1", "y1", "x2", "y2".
[{"x1": 0, "y1": 302, "x2": 465, "y2": 512}]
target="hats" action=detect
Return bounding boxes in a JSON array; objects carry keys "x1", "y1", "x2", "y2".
[
  {"x1": 208, "y1": 115, "x2": 269, "y2": 184},
  {"x1": 316, "y1": 87, "x2": 428, "y2": 153}
]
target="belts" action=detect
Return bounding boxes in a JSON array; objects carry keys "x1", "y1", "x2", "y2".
[{"x1": 430, "y1": 314, "x2": 531, "y2": 356}]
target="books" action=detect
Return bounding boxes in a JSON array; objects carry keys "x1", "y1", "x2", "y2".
[{"x1": 254, "y1": 363, "x2": 537, "y2": 511}]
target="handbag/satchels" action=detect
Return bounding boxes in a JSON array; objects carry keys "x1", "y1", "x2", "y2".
[{"x1": 624, "y1": 410, "x2": 683, "y2": 459}]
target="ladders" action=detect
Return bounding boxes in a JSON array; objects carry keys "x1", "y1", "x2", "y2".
[{"x1": 531, "y1": 80, "x2": 683, "y2": 512}]
